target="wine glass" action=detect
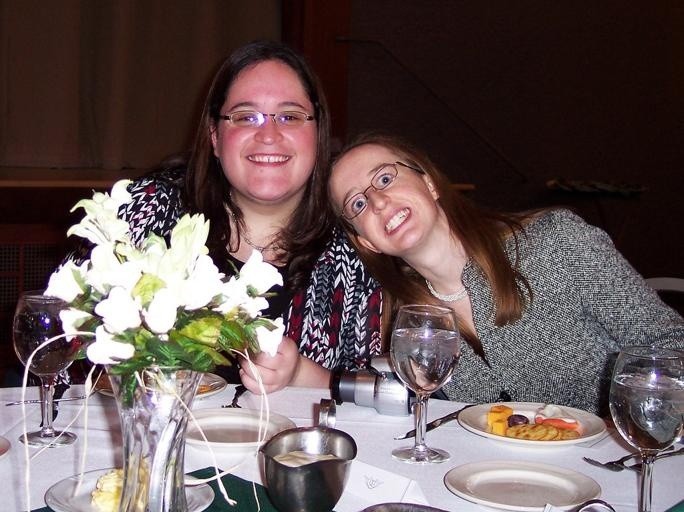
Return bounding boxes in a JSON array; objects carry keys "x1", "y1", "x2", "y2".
[
  {"x1": 389, "y1": 304, "x2": 462, "y2": 464},
  {"x1": 608, "y1": 346, "x2": 684, "y2": 512},
  {"x1": 13, "y1": 294, "x2": 81, "y2": 448}
]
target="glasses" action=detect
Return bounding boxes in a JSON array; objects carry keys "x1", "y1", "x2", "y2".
[
  {"x1": 221, "y1": 107, "x2": 318, "y2": 129},
  {"x1": 336, "y1": 159, "x2": 426, "y2": 220}
]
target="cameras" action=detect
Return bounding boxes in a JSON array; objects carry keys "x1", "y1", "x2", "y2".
[{"x1": 329, "y1": 358, "x2": 414, "y2": 416}]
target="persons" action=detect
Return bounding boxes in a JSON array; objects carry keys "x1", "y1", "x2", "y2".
[
  {"x1": 324, "y1": 127, "x2": 682, "y2": 422},
  {"x1": 78, "y1": 40, "x2": 384, "y2": 396}
]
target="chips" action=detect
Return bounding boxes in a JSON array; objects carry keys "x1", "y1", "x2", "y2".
[{"x1": 505, "y1": 423, "x2": 580, "y2": 441}]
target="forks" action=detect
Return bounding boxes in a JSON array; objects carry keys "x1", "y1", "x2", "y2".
[{"x1": 582, "y1": 442, "x2": 684, "y2": 472}]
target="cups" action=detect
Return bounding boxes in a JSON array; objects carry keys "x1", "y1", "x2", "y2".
[{"x1": 257, "y1": 396, "x2": 359, "y2": 512}]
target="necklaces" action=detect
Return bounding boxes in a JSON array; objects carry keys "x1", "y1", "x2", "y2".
[
  {"x1": 228, "y1": 199, "x2": 292, "y2": 252},
  {"x1": 424, "y1": 277, "x2": 467, "y2": 303}
]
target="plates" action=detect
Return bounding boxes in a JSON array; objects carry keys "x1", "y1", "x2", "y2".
[
  {"x1": 443, "y1": 459, "x2": 601, "y2": 512},
  {"x1": 165, "y1": 406, "x2": 297, "y2": 453},
  {"x1": 44, "y1": 465, "x2": 216, "y2": 512},
  {"x1": 92, "y1": 370, "x2": 228, "y2": 399},
  {"x1": 457, "y1": 399, "x2": 608, "y2": 448}
]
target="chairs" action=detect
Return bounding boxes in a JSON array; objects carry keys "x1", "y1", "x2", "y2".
[{"x1": 643, "y1": 274, "x2": 683, "y2": 317}]
